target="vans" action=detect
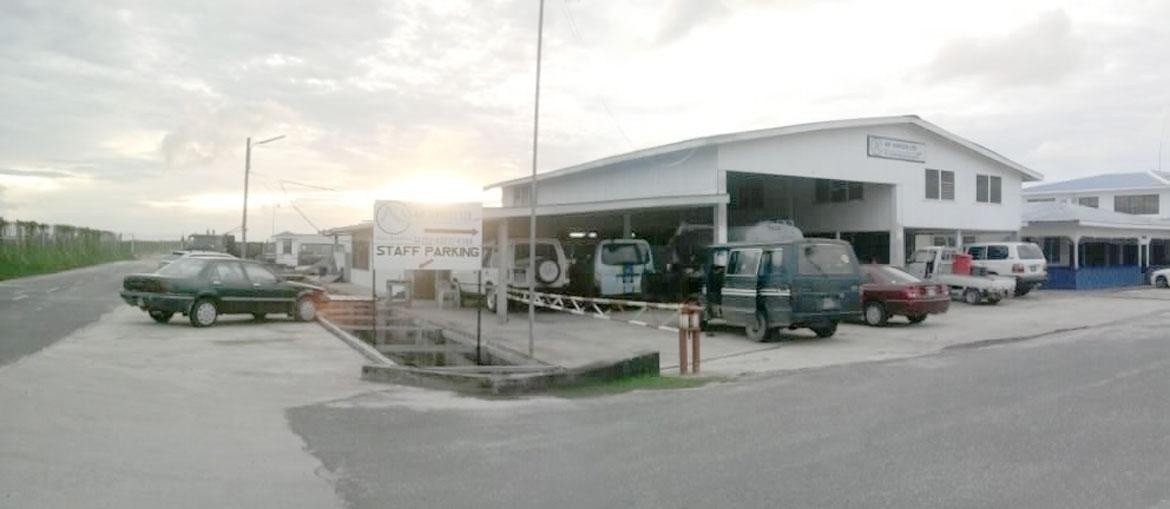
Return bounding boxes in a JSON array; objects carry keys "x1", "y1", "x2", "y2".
[
  {"x1": 685, "y1": 238, "x2": 864, "y2": 343},
  {"x1": 570, "y1": 240, "x2": 655, "y2": 297},
  {"x1": 961, "y1": 241, "x2": 1048, "y2": 296}
]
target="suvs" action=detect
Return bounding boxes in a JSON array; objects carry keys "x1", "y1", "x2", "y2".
[{"x1": 449, "y1": 236, "x2": 567, "y2": 312}]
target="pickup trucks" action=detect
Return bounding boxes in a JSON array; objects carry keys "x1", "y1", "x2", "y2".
[{"x1": 902, "y1": 244, "x2": 1017, "y2": 305}]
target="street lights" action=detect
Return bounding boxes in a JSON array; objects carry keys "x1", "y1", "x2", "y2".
[{"x1": 240, "y1": 134, "x2": 286, "y2": 256}]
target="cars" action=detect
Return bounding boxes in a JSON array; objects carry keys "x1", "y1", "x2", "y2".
[
  {"x1": 860, "y1": 262, "x2": 950, "y2": 327},
  {"x1": 119, "y1": 254, "x2": 331, "y2": 325},
  {"x1": 1150, "y1": 266, "x2": 1170, "y2": 289}
]
